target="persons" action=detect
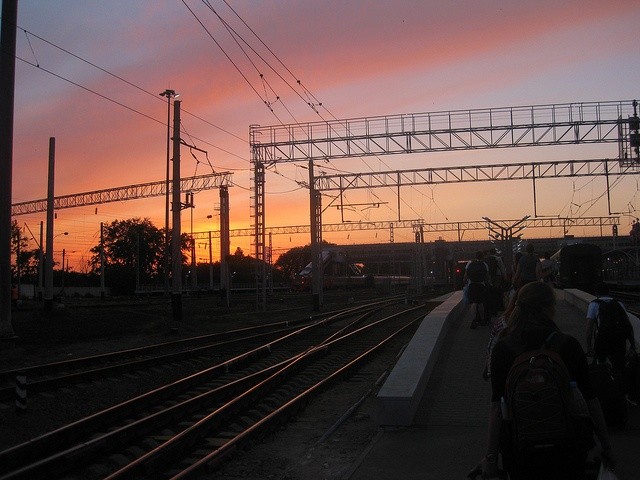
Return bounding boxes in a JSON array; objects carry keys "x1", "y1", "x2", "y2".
[
  {"x1": 484, "y1": 288, "x2": 520, "y2": 392},
  {"x1": 470, "y1": 282, "x2": 617, "y2": 480},
  {"x1": 463, "y1": 251, "x2": 489, "y2": 329},
  {"x1": 585, "y1": 283, "x2": 634, "y2": 427},
  {"x1": 511, "y1": 244, "x2": 542, "y2": 287},
  {"x1": 482, "y1": 247, "x2": 506, "y2": 314},
  {"x1": 542, "y1": 252, "x2": 559, "y2": 282}
]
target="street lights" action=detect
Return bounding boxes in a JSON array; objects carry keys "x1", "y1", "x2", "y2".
[{"x1": 482, "y1": 216, "x2": 530, "y2": 256}]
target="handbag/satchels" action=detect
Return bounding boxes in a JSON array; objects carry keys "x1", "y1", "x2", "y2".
[
  {"x1": 536, "y1": 257, "x2": 545, "y2": 278},
  {"x1": 621, "y1": 347, "x2": 640, "y2": 406}
]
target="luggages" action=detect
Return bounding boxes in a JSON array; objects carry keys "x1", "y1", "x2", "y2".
[{"x1": 584, "y1": 352, "x2": 628, "y2": 431}]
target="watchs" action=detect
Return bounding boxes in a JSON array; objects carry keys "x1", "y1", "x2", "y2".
[{"x1": 482, "y1": 454, "x2": 500, "y2": 466}]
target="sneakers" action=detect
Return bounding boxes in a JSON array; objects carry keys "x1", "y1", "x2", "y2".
[
  {"x1": 479, "y1": 319, "x2": 487, "y2": 327},
  {"x1": 470, "y1": 320, "x2": 479, "y2": 330}
]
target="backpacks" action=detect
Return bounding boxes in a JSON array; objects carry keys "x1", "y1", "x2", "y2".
[
  {"x1": 466, "y1": 260, "x2": 487, "y2": 282},
  {"x1": 592, "y1": 297, "x2": 631, "y2": 348},
  {"x1": 500, "y1": 328, "x2": 597, "y2": 463},
  {"x1": 482, "y1": 255, "x2": 497, "y2": 275}
]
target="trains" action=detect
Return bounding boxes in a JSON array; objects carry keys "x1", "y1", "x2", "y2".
[
  {"x1": 550, "y1": 243, "x2": 602, "y2": 288},
  {"x1": 298, "y1": 252, "x2": 410, "y2": 288},
  {"x1": 448, "y1": 261, "x2": 471, "y2": 288}
]
